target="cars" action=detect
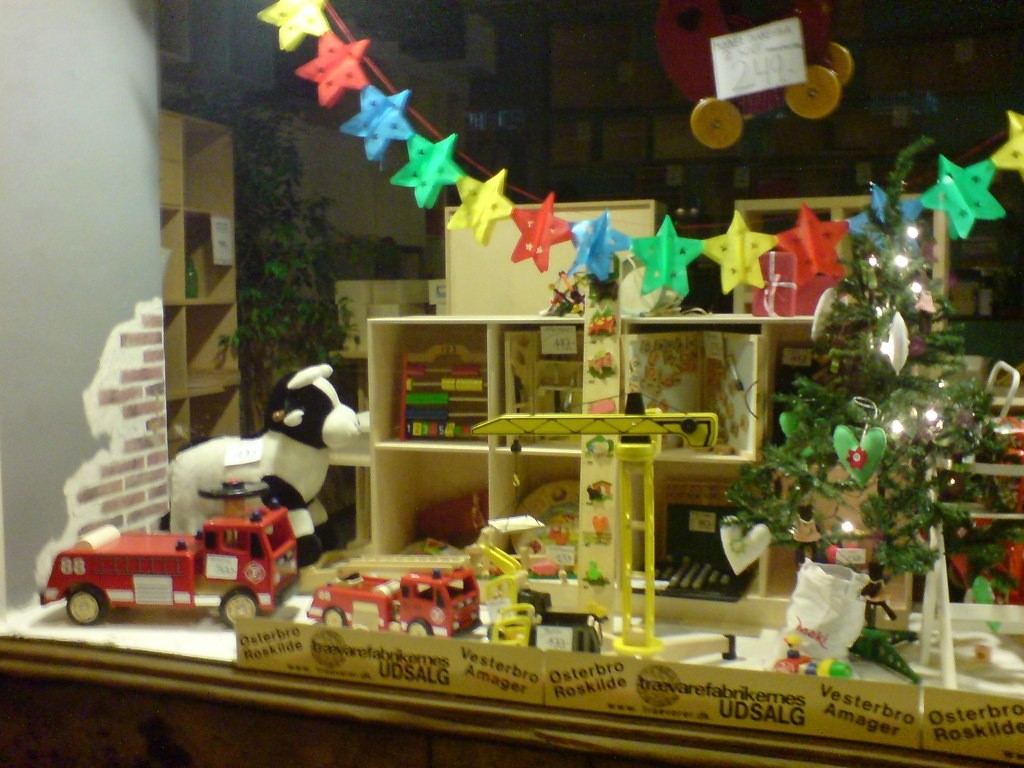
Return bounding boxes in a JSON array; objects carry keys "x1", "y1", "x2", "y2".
[{"x1": 651, "y1": 0, "x2": 856, "y2": 151}]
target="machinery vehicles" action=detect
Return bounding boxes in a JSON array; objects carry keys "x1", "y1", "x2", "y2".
[{"x1": 468, "y1": 515, "x2": 610, "y2": 655}]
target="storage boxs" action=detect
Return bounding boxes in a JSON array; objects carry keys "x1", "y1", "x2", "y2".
[{"x1": 549, "y1": 0, "x2": 1024, "y2": 235}]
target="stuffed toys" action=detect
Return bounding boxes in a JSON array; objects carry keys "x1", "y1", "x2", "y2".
[{"x1": 168, "y1": 364, "x2": 360, "y2": 567}]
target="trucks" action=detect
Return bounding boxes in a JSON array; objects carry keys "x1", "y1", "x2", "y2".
[
  {"x1": 34, "y1": 474, "x2": 300, "y2": 630},
  {"x1": 307, "y1": 570, "x2": 482, "y2": 640}
]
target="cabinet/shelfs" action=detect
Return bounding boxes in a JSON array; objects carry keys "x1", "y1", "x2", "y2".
[
  {"x1": 365, "y1": 311, "x2": 933, "y2": 629},
  {"x1": 160, "y1": 107, "x2": 242, "y2": 456}
]
